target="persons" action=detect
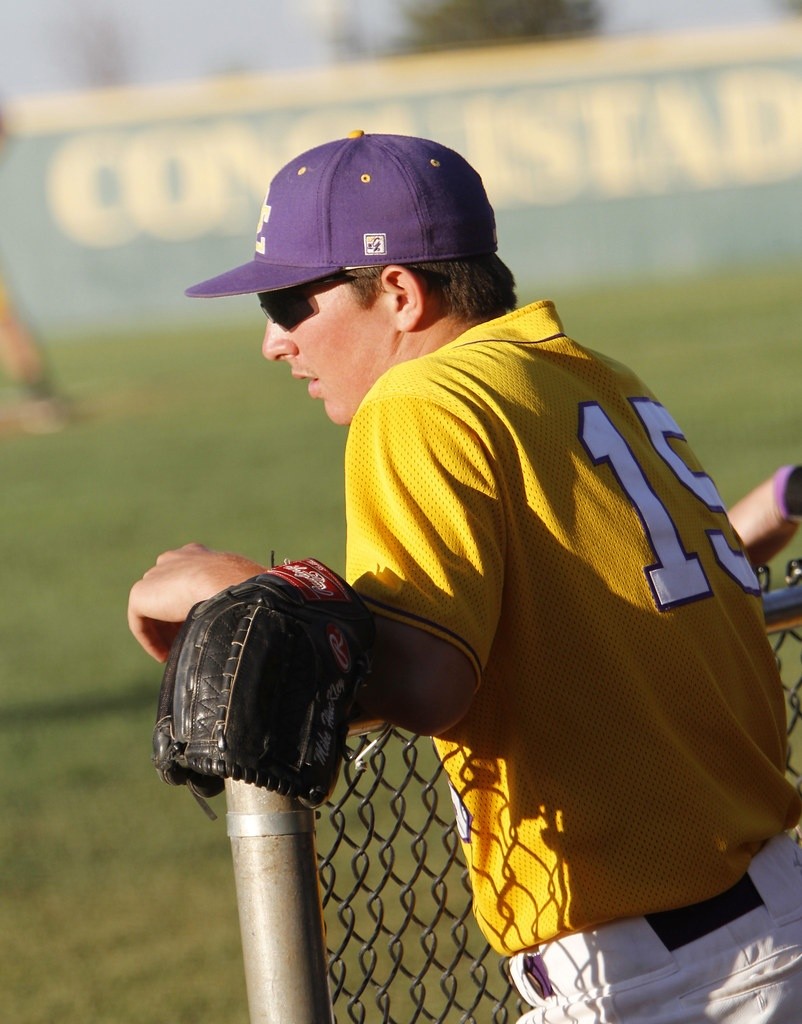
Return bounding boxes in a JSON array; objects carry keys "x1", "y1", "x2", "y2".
[
  {"x1": 0, "y1": 103, "x2": 76, "y2": 438},
  {"x1": 129, "y1": 129, "x2": 802, "y2": 1024}
]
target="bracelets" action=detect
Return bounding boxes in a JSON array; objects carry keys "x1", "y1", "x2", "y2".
[{"x1": 773, "y1": 464, "x2": 796, "y2": 522}]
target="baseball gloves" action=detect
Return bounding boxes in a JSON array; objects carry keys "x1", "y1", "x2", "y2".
[{"x1": 148, "y1": 548, "x2": 390, "y2": 827}]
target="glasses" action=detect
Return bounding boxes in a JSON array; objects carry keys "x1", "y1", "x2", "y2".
[{"x1": 260, "y1": 261, "x2": 403, "y2": 330}]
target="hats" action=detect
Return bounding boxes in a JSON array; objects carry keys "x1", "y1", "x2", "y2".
[{"x1": 182, "y1": 129, "x2": 499, "y2": 299}]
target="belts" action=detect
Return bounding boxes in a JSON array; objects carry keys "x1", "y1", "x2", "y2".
[{"x1": 508, "y1": 872, "x2": 762, "y2": 1008}]
